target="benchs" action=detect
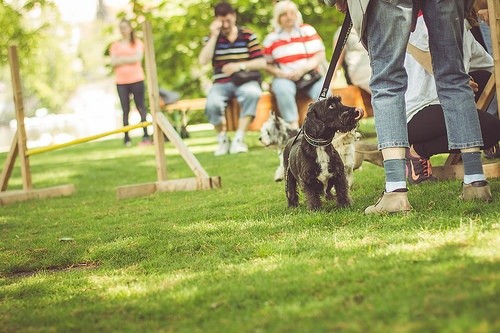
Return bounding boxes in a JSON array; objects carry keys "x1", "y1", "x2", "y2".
[
  {"x1": 161, "y1": 98, "x2": 207, "y2": 131},
  {"x1": 218, "y1": 85, "x2": 373, "y2": 131}
]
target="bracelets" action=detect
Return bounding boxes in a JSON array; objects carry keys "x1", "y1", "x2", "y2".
[{"x1": 239, "y1": 62, "x2": 245, "y2": 71}]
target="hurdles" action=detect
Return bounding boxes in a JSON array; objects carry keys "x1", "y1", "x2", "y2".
[{"x1": 0, "y1": 22, "x2": 221, "y2": 206}]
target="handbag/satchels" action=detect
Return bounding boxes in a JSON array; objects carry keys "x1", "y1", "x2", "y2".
[
  {"x1": 231, "y1": 70, "x2": 260, "y2": 87},
  {"x1": 296, "y1": 69, "x2": 321, "y2": 91}
]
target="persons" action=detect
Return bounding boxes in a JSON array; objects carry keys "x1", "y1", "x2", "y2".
[
  {"x1": 333, "y1": 25, "x2": 372, "y2": 97},
  {"x1": 109, "y1": 19, "x2": 147, "y2": 145},
  {"x1": 264, "y1": 0, "x2": 333, "y2": 132},
  {"x1": 325, "y1": 0, "x2": 494, "y2": 215},
  {"x1": 199, "y1": 2, "x2": 266, "y2": 156},
  {"x1": 403, "y1": 15, "x2": 500, "y2": 183}
]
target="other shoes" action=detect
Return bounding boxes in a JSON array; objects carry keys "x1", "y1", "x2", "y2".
[
  {"x1": 214, "y1": 140, "x2": 229, "y2": 156},
  {"x1": 285, "y1": 127, "x2": 302, "y2": 144},
  {"x1": 274, "y1": 153, "x2": 285, "y2": 181},
  {"x1": 228, "y1": 139, "x2": 249, "y2": 154}
]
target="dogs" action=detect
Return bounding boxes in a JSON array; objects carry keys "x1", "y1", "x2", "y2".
[{"x1": 259, "y1": 95, "x2": 364, "y2": 211}]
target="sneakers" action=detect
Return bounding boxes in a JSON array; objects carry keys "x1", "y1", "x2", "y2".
[
  {"x1": 364, "y1": 188, "x2": 414, "y2": 215},
  {"x1": 405, "y1": 146, "x2": 436, "y2": 186},
  {"x1": 457, "y1": 179, "x2": 493, "y2": 204}
]
